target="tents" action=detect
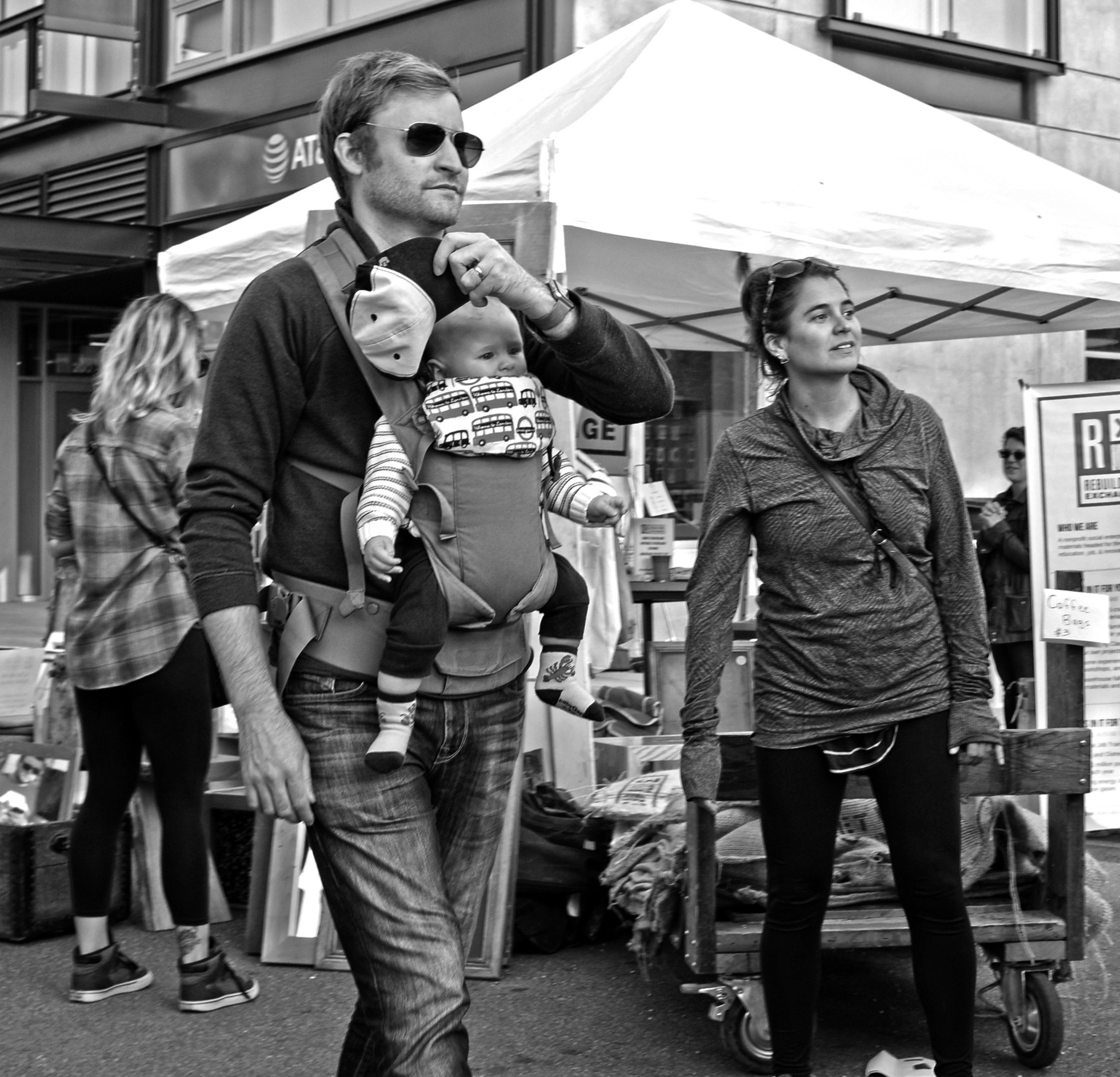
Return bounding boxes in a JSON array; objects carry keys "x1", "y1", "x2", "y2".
[{"x1": 155, "y1": 0, "x2": 1120, "y2": 916}]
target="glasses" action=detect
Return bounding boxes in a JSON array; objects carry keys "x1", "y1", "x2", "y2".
[
  {"x1": 762, "y1": 257, "x2": 840, "y2": 333},
  {"x1": 999, "y1": 449, "x2": 1025, "y2": 460},
  {"x1": 363, "y1": 122, "x2": 485, "y2": 169},
  {"x1": 194, "y1": 349, "x2": 211, "y2": 378}
]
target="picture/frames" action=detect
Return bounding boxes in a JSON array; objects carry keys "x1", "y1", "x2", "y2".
[{"x1": 242, "y1": 735, "x2": 524, "y2": 979}]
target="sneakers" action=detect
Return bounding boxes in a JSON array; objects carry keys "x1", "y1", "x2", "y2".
[
  {"x1": 68, "y1": 942, "x2": 154, "y2": 1002},
  {"x1": 177, "y1": 938, "x2": 259, "y2": 1012}
]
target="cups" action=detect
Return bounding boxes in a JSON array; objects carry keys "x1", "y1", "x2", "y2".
[{"x1": 652, "y1": 554, "x2": 671, "y2": 583}]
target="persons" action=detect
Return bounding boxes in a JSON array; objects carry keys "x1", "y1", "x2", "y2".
[
  {"x1": 679, "y1": 258, "x2": 1004, "y2": 1076},
  {"x1": 357, "y1": 296, "x2": 629, "y2": 774},
  {"x1": 42, "y1": 296, "x2": 260, "y2": 1014},
  {"x1": 977, "y1": 426, "x2": 1033, "y2": 728},
  {"x1": 178, "y1": 54, "x2": 674, "y2": 1077}
]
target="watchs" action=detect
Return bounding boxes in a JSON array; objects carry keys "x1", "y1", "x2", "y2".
[{"x1": 528, "y1": 278, "x2": 575, "y2": 331}]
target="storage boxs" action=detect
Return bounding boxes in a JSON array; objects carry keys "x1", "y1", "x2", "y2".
[{"x1": 0, "y1": 800, "x2": 134, "y2": 946}]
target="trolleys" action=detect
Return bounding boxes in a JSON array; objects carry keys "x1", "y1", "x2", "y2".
[{"x1": 588, "y1": 550, "x2": 1093, "y2": 1077}]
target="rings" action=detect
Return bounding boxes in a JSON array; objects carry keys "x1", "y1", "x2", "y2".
[{"x1": 473, "y1": 265, "x2": 484, "y2": 282}]
